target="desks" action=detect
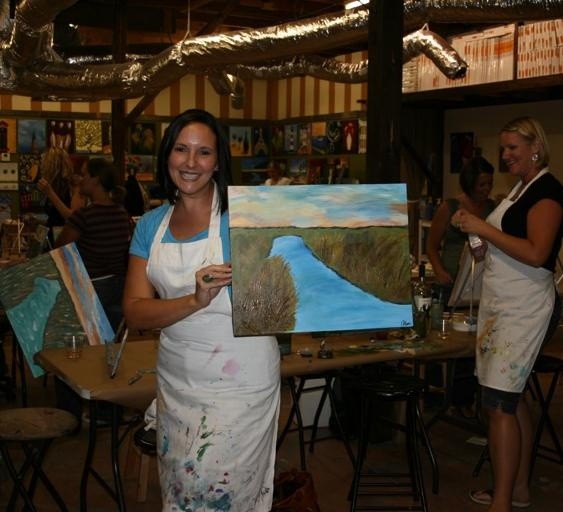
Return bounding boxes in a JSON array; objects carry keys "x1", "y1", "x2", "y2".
[{"x1": 34, "y1": 328, "x2": 476, "y2": 512}]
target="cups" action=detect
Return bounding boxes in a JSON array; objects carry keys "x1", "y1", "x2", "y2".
[
  {"x1": 434, "y1": 318, "x2": 451, "y2": 342},
  {"x1": 60, "y1": 331, "x2": 87, "y2": 361},
  {"x1": 297, "y1": 346, "x2": 312, "y2": 372},
  {"x1": 104, "y1": 335, "x2": 130, "y2": 384}
]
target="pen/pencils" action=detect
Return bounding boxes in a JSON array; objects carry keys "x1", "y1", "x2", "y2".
[
  {"x1": 11, "y1": 215, "x2": 25, "y2": 257},
  {"x1": 128, "y1": 375, "x2": 143, "y2": 386},
  {"x1": 109, "y1": 327, "x2": 130, "y2": 378}
]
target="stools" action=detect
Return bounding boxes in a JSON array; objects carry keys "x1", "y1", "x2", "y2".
[
  {"x1": 473, "y1": 354, "x2": 563, "y2": 486},
  {"x1": 276, "y1": 369, "x2": 355, "y2": 471},
  {"x1": 132, "y1": 425, "x2": 157, "y2": 455},
  {"x1": 0, "y1": 407, "x2": 78, "y2": 512},
  {"x1": 347, "y1": 375, "x2": 429, "y2": 512}
]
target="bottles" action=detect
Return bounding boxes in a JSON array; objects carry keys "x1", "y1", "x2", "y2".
[
  {"x1": 409, "y1": 260, "x2": 433, "y2": 333},
  {"x1": 461, "y1": 213, "x2": 487, "y2": 262}
]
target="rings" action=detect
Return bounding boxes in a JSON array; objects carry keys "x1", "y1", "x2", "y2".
[{"x1": 201, "y1": 275, "x2": 212, "y2": 282}]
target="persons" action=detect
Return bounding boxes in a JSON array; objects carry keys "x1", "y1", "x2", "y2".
[
  {"x1": 37, "y1": 148, "x2": 92, "y2": 243},
  {"x1": 54, "y1": 159, "x2": 135, "y2": 437},
  {"x1": 426, "y1": 156, "x2": 506, "y2": 420},
  {"x1": 450, "y1": 115, "x2": 562, "y2": 512},
  {"x1": 264, "y1": 161, "x2": 293, "y2": 186},
  {"x1": 122, "y1": 108, "x2": 281, "y2": 512}
]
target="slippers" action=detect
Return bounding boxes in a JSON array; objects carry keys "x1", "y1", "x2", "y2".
[{"x1": 467, "y1": 484, "x2": 534, "y2": 508}]
